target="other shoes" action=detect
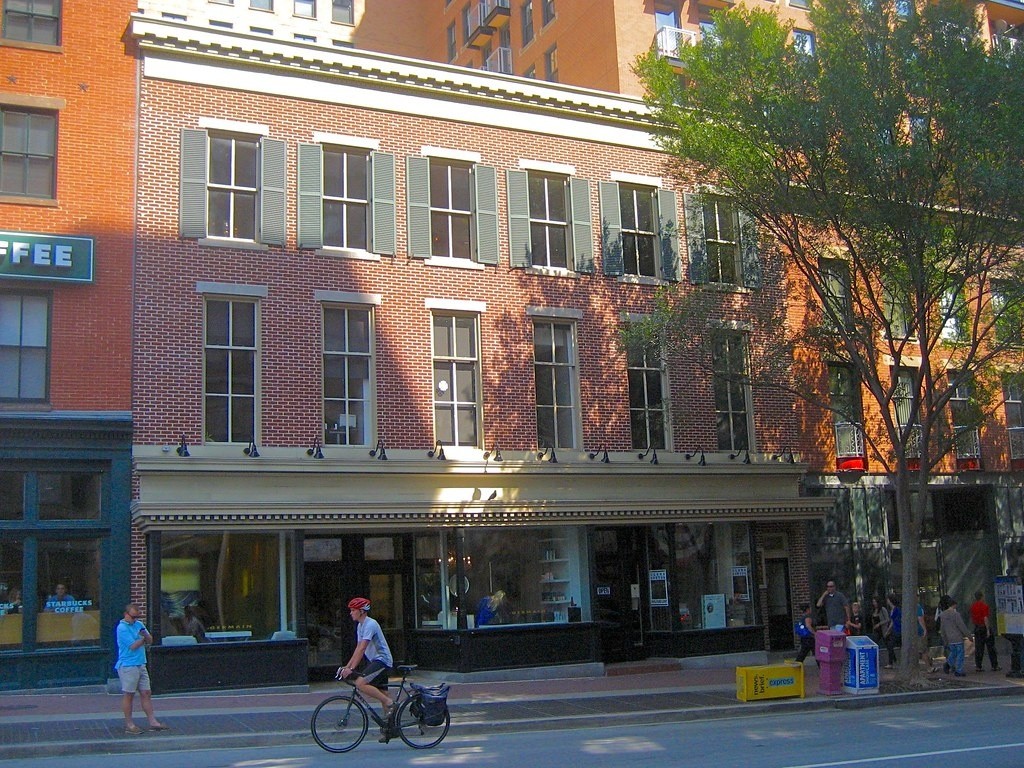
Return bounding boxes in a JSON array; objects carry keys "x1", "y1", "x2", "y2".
[
  {"x1": 992, "y1": 666, "x2": 1002, "y2": 671},
  {"x1": 944, "y1": 662, "x2": 950, "y2": 674},
  {"x1": 976, "y1": 667, "x2": 985, "y2": 671},
  {"x1": 383, "y1": 700, "x2": 399, "y2": 719},
  {"x1": 379, "y1": 733, "x2": 399, "y2": 743},
  {"x1": 125, "y1": 726, "x2": 145, "y2": 734},
  {"x1": 149, "y1": 723, "x2": 169, "y2": 731},
  {"x1": 955, "y1": 672, "x2": 966, "y2": 676},
  {"x1": 928, "y1": 668, "x2": 939, "y2": 673},
  {"x1": 885, "y1": 665, "x2": 894, "y2": 669}
]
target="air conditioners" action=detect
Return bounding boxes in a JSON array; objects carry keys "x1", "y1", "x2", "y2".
[{"x1": 485, "y1": 47, "x2": 512, "y2": 75}]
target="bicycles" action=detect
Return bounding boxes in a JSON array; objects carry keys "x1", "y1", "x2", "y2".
[{"x1": 311, "y1": 665, "x2": 451, "y2": 754}]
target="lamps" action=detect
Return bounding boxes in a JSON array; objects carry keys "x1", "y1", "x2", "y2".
[
  {"x1": 729, "y1": 445, "x2": 751, "y2": 464},
  {"x1": 483, "y1": 441, "x2": 503, "y2": 461},
  {"x1": 439, "y1": 534, "x2": 471, "y2": 575},
  {"x1": 686, "y1": 445, "x2": 706, "y2": 466},
  {"x1": 244, "y1": 436, "x2": 260, "y2": 458},
  {"x1": 369, "y1": 440, "x2": 388, "y2": 460},
  {"x1": 538, "y1": 443, "x2": 558, "y2": 463},
  {"x1": 590, "y1": 443, "x2": 610, "y2": 463},
  {"x1": 306, "y1": 436, "x2": 325, "y2": 459},
  {"x1": 638, "y1": 444, "x2": 658, "y2": 464},
  {"x1": 428, "y1": 440, "x2": 446, "y2": 460},
  {"x1": 772, "y1": 446, "x2": 794, "y2": 464},
  {"x1": 177, "y1": 435, "x2": 190, "y2": 457}
]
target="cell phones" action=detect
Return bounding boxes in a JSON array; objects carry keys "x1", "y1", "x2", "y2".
[{"x1": 140, "y1": 628, "x2": 145, "y2": 632}]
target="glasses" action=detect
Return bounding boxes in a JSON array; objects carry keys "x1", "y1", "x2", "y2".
[
  {"x1": 826, "y1": 586, "x2": 834, "y2": 588},
  {"x1": 128, "y1": 613, "x2": 140, "y2": 619}
]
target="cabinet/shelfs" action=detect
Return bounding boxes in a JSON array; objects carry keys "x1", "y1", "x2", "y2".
[{"x1": 535, "y1": 535, "x2": 572, "y2": 622}]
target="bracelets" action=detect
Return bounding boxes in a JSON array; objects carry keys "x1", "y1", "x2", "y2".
[{"x1": 349, "y1": 667, "x2": 354, "y2": 672}]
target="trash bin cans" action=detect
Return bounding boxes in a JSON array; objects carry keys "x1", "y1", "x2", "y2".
[
  {"x1": 843, "y1": 636, "x2": 879, "y2": 695},
  {"x1": 736, "y1": 660, "x2": 806, "y2": 702}
]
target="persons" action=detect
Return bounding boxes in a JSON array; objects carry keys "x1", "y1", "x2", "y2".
[
  {"x1": 871, "y1": 596, "x2": 898, "y2": 664},
  {"x1": 884, "y1": 594, "x2": 902, "y2": 668},
  {"x1": 43, "y1": 584, "x2": 78, "y2": 648},
  {"x1": 796, "y1": 581, "x2": 863, "y2": 669},
  {"x1": 935, "y1": 595, "x2": 973, "y2": 676},
  {"x1": 0, "y1": 589, "x2": 21, "y2": 613},
  {"x1": 473, "y1": 590, "x2": 506, "y2": 625},
  {"x1": 917, "y1": 596, "x2": 938, "y2": 674},
  {"x1": 969, "y1": 592, "x2": 1002, "y2": 672},
  {"x1": 114, "y1": 605, "x2": 170, "y2": 734},
  {"x1": 341, "y1": 598, "x2": 398, "y2": 743},
  {"x1": 183, "y1": 606, "x2": 205, "y2": 642}
]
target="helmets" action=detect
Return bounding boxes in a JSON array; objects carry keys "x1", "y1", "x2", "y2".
[{"x1": 348, "y1": 598, "x2": 371, "y2": 610}]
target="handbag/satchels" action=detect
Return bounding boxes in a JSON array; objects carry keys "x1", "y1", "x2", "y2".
[
  {"x1": 412, "y1": 681, "x2": 450, "y2": 727},
  {"x1": 794, "y1": 616, "x2": 814, "y2": 638}
]
